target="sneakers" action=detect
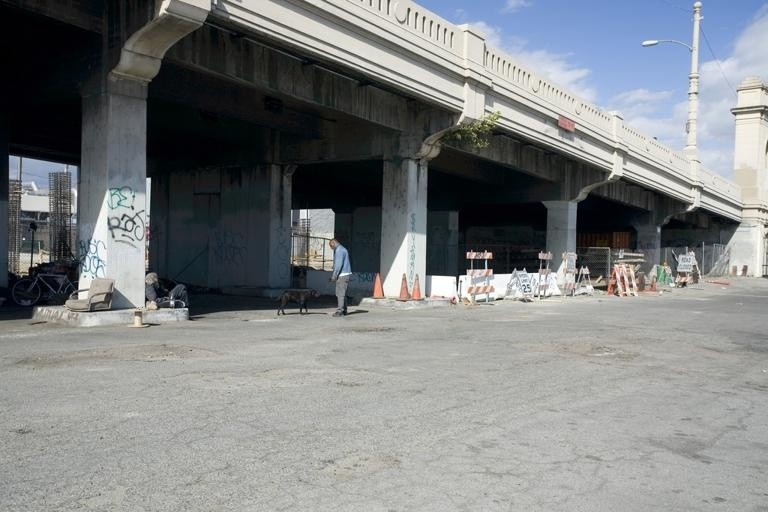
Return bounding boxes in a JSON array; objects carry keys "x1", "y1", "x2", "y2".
[{"x1": 331, "y1": 307, "x2": 347, "y2": 318}]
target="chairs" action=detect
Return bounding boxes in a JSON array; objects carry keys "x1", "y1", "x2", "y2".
[{"x1": 63, "y1": 275, "x2": 115, "y2": 313}]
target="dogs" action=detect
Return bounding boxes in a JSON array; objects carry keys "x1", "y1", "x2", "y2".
[{"x1": 270, "y1": 289, "x2": 321, "y2": 315}]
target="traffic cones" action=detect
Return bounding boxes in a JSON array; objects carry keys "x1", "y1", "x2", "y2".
[
  {"x1": 649, "y1": 276, "x2": 657, "y2": 292},
  {"x1": 396, "y1": 273, "x2": 411, "y2": 301},
  {"x1": 372, "y1": 272, "x2": 385, "y2": 299},
  {"x1": 412, "y1": 274, "x2": 423, "y2": 300}
]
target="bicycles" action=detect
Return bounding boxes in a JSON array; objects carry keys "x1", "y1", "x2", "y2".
[{"x1": 12, "y1": 266, "x2": 78, "y2": 307}]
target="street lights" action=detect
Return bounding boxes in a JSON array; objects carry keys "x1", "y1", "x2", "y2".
[{"x1": 642, "y1": 2, "x2": 703, "y2": 161}]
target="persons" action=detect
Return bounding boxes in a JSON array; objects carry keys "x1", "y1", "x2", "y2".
[
  {"x1": 327, "y1": 238, "x2": 352, "y2": 317},
  {"x1": 146, "y1": 272, "x2": 191, "y2": 308}
]
